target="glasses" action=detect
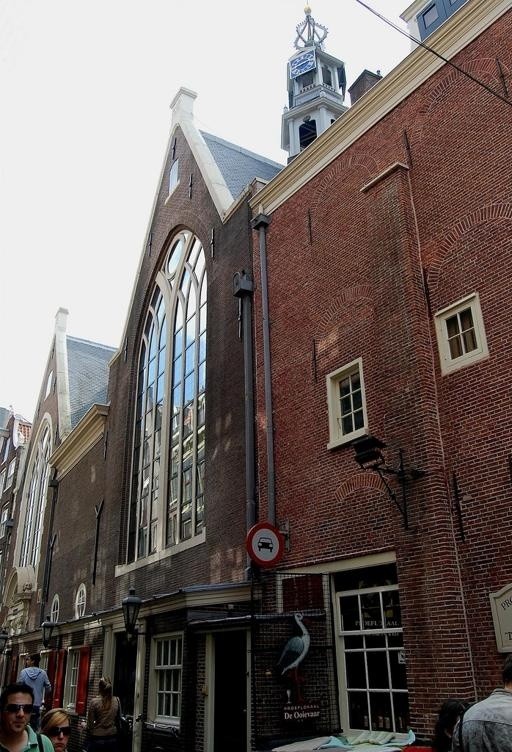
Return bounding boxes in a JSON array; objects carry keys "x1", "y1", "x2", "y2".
[
  {"x1": 6, "y1": 704, "x2": 33, "y2": 713},
  {"x1": 48, "y1": 726, "x2": 70, "y2": 736}
]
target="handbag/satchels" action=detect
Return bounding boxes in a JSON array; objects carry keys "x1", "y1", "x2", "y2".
[{"x1": 115, "y1": 712, "x2": 129, "y2": 736}]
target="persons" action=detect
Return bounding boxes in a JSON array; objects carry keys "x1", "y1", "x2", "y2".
[
  {"x1": 17, "y1": 653, "x2": 52, "y2": 733},
  {"x1": 81, "y1": 675, "x2": 124, "y2": 752},
  {"x1": 38, "y1": 707, "x2": 73, "y2": 752},
  {"x1": 451, "y1": 658, "x2": 512, "y2": 752},
  {"x1": 0, "y1": 681, "x2": 55, "y2": 752},
  {"x1": 431, "y1": 699, "x2": 471, "y2": 752}
]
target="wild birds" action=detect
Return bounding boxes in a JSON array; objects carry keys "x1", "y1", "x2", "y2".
[{"x1": 275, "y1": 613, "x2": 313, "y2": 705}]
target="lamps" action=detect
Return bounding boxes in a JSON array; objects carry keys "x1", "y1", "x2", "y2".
[
  {"x1": 352, "y1": 435, "x2": 417, "y2": 529},
  {"x1": 123, "y1": 587, "x2": 150, "y2": 644},
  {"x1": 41, "y1": 615, "x2": 59, "y2": 648},
  {"x1": 0, "y1": 629, "x2": 10, "y2": 656}
]
